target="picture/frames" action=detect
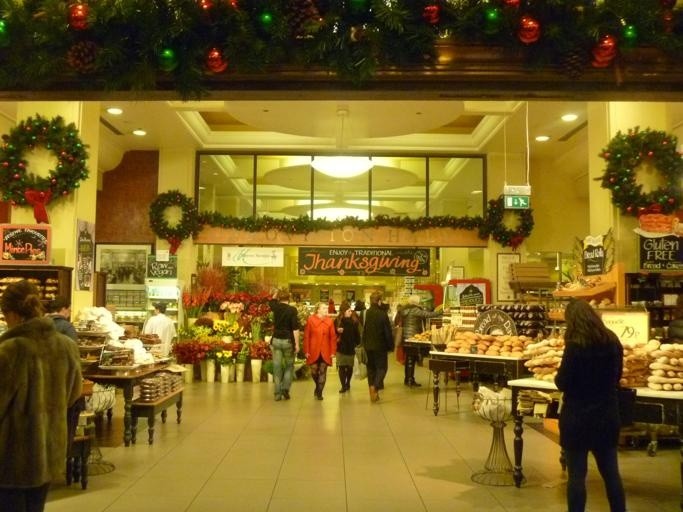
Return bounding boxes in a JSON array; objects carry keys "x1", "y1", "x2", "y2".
[{"x1": 90, "y1": 241, "x2": 153, "y2": 276}]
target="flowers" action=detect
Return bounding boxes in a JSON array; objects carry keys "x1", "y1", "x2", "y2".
[{"x1": 175, "y1": 269, "x2": 282, "y2": 390}]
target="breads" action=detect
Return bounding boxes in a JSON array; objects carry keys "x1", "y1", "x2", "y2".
[{"x1": 407, "y1": 328, "x2": 683, "y2": 391}]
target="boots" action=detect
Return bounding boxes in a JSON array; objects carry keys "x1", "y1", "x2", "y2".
[
  {"x1": 404, "y1": 377, "x2": 421, "y2": 387},
  {"x1": 369, "y1": 384, "x2": 379, "y2": 402}
]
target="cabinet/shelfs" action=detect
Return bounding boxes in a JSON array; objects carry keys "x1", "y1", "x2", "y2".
[
  {"x1": 506, "y1": 279, "x2": 569, "y2": 325},
  {"x1": 0, "y1": 263, "x2": 78, "y2": 328},
  {"x1": 549, "y1": 267, "x2": 619, "y2": 328},
  {"x1": 625, "y1": 270, "x2": 682, "y2": 326},
  {"x1": 142, "y1": 277, "x2": 184, "y2": 352}
]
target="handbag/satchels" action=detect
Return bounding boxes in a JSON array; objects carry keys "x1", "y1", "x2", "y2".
[
  {"x1": 394, "y1": 326, "x2": 403, "y2": 346},
  {"x1": 620, "y1": 388, "x2": 637, "y2": 425},
  {"x1": 270, "y1": 336, "x2": 273, "y2": 345},
  {"x1": 384, "y1": 324, "x2": 394, "y2": 352},
  {"x1": 355, "y1": 344, "x2": 368, "y2": 380},
  {"x1": 397, "y1": 342, "x2": 407, "y2": 364},
  {"x1": 279, "y1": 330, "x2": 290, "y2": 339}
]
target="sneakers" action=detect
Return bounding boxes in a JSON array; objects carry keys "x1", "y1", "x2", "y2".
[
  {"x1": 274, "y1": 392, "x2": 282, "y2": 401},
  {"x1": 282, "y1": 389, "x2": 290, "y2": 399}
]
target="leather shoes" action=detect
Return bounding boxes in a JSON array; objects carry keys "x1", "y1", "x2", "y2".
[
  {"x1": 339, "y1": 384, "x2": 350, "y2": 392},
  {"x1": 314, "y1": 390, "x2": 323, "y2": 400}
]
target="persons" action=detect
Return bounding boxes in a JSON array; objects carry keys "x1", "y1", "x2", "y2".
[
  {"x1": 301, "y1": 300, "x2": 337, "y2": 402},
  {"x1": 0, "y1": 277, "x2": 85, "y2": 511},
  {"x1": 401, "y1": 294, "x2": 451, "y2": 390},
  {"x1": 332, "y1": 300, "x2": 360, "y2": 394},
  {"x1": 140, "y1": 301, "x2": 178, "y2": 357},
  {"x1": 267, "y1": 287, "x2": 302, "y2": 402},
  {"x1": 362, "y1": 291, "x2": 397, "y2": 406},
  {"x1": 41, "y1": 293, "x2": 79, "y2": 345},
  {"x1": 392, "y1": 301, "x2": 402, "y2": 328},
  {"x1": 99, "y1": 248, "x2": 144, "y2": 284},
  {"x1": 553, "y1": 297, "x2": 628, "y2": 511}
]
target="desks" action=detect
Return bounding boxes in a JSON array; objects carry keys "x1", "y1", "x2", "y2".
[
  {"x1": 130, "y1": 388, "x2": 185, "y2": 447},
  {"x1": 76, "y1": 362, "x2": 172, "y2": 448}
]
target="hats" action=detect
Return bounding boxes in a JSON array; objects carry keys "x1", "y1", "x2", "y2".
[
  {"x1": 407, "y1": 295, "x2": 420, "y2": 306},
  {"x1": 152, "y1": 303, "x2": 166, "y2": 308}
]
